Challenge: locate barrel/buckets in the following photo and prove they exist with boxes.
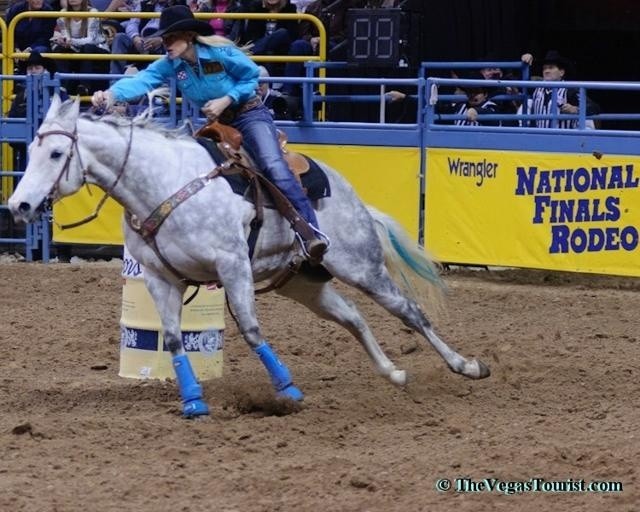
[119,247,225,380]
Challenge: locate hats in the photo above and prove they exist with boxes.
[535,48,566,66]
[17,51,56,70]
[101,19,126,33]
[144,3,215,40]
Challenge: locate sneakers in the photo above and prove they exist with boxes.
[305,236,325,267]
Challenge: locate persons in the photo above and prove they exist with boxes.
[87,4,332,268]
[1,0,595,136]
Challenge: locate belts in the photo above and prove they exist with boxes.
[220,96,261,119]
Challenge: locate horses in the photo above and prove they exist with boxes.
[7,88,491,420]
